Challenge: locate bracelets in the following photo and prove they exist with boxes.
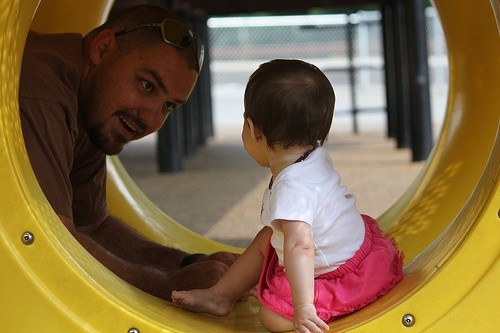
[181,252,208,268]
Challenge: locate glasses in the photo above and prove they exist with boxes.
[113,18,205,76]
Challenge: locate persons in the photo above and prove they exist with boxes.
[18,0,243,302]
[171,59,405,333]
